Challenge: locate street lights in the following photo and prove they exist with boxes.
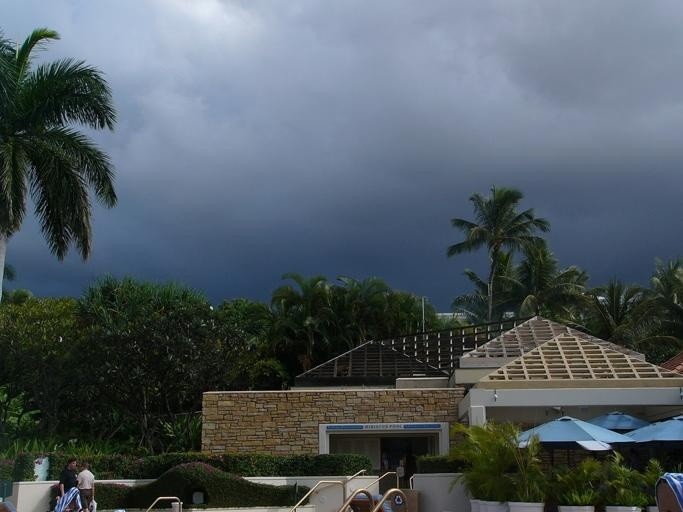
[89,500,97,512]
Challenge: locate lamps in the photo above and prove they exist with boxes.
[1,500,17,512]
[54,486,83,512]
[655,473,682,512]
[347,493,393,512]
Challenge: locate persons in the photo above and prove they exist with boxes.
[380,452,389,470]
[77,462,96,512]
[58,457,79,512]
[396,458,405,488]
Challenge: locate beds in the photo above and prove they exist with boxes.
[512,409,683,473]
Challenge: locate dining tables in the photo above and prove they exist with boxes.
[447,415,667,512]
